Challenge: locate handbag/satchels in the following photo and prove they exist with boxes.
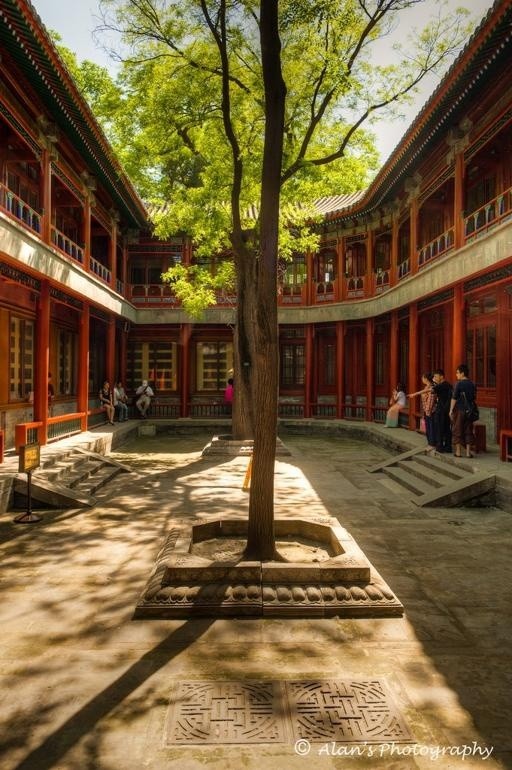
[463,401,479,422]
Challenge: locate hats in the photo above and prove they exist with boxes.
[142,380,148,386]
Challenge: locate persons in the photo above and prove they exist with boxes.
[222,379,234,411]
[135,381,155,417]
[96,380,115,424]
[47,371,56,417]
[113,380,131,422]
[405,364,480,460]
[383,381,407,430]
